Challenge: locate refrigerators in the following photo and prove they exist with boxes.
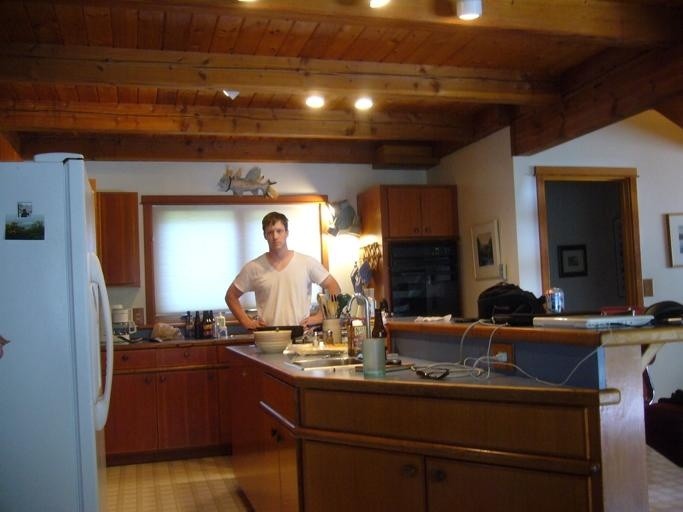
[0,160,112,508]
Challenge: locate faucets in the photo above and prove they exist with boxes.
[347,295,370,339]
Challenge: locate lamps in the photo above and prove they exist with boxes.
[456,1,482,22]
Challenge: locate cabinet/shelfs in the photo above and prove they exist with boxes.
[102,367,219,455]
[260,410,300,511]
[157,346,217,366]
[301,441,593,512]
[387,188,457,239]
[95,192,141,285]
[100,348,157,372]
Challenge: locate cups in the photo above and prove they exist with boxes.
[322,319,342,346]
[363,338,388,377]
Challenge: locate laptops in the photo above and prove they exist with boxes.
[532,313,657,331]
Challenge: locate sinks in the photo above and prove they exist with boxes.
[284,356,363,372]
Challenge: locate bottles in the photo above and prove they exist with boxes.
[202,310,214,338]
[325,330,334,346]
[195,311,201,339]
[312,332,319,347]
[371,308,389,362]
[184,311,193,339]
[545,286,564,313]
[217,326,227,339]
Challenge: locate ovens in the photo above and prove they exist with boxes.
[389,245,459,319]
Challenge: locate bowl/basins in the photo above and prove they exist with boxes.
[254,328,290,354]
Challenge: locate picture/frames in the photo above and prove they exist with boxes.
[470,217,502,281]
[558,245,587,278]
[666,213,683,267]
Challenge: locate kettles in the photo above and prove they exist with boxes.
[111,304,137,345]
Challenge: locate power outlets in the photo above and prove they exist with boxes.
[491,343,514,371]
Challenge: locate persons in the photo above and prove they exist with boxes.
[224,212,341,334]
